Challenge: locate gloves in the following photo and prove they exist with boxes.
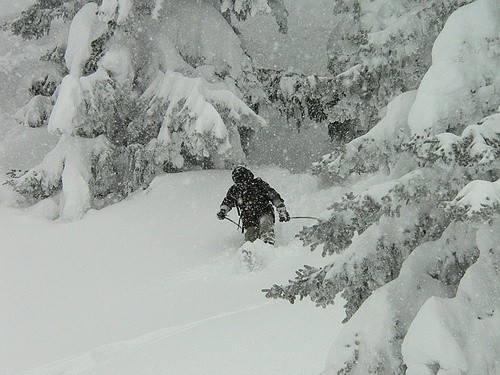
[276,207,290,222]
[217,204,231,220]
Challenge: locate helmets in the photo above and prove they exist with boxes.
[232,166,251,183]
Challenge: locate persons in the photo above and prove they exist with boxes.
[217,166,290,246]
